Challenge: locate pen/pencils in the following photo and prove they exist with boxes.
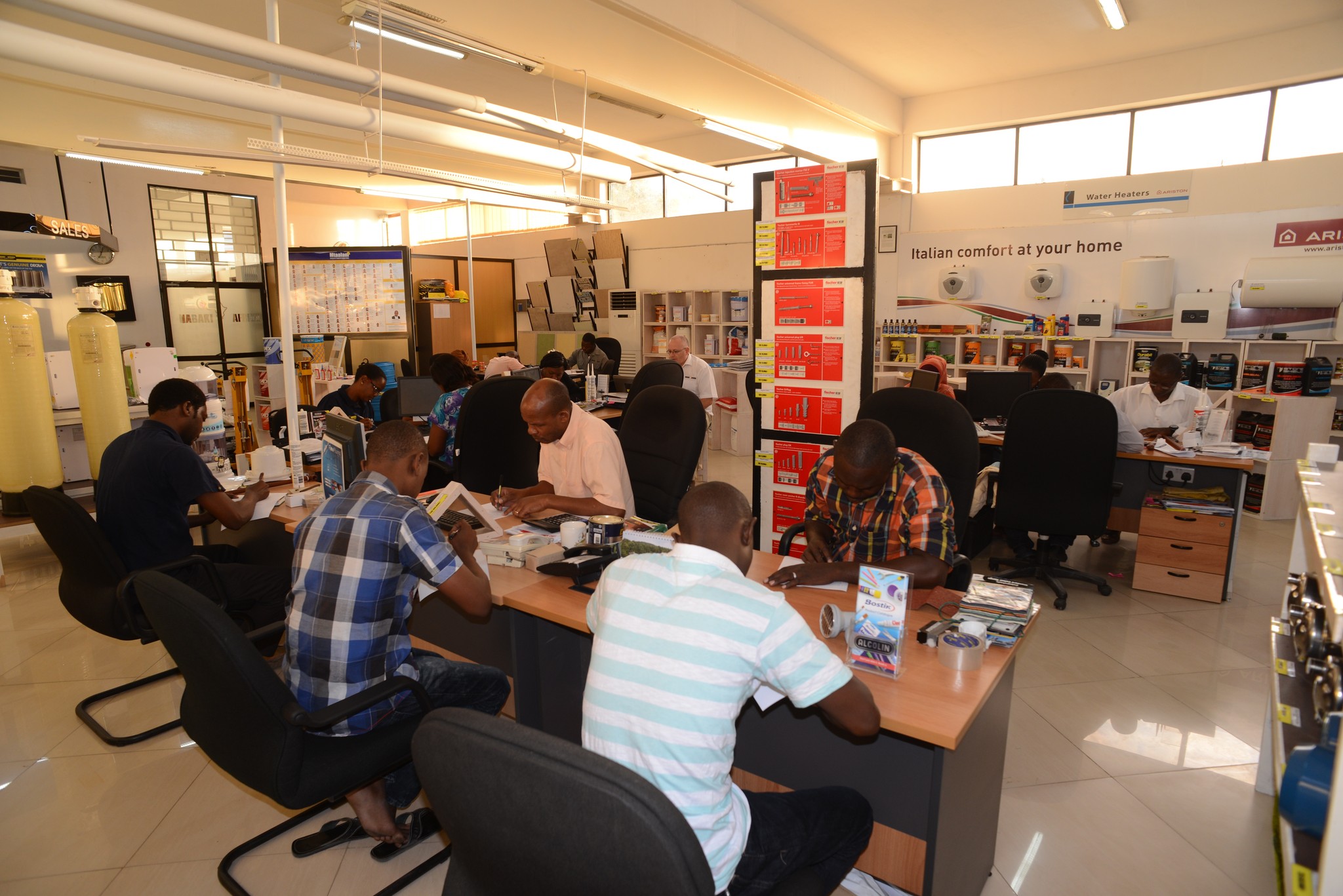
[448,527,462,540]
[496,485,501,512]
[528,364,532,368]
[259,472,264,481]
[353,412,375,427]
[820,537,836,560]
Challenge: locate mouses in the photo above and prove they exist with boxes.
[426,494,439,504]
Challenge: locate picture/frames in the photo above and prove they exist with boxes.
[878,224,897,253]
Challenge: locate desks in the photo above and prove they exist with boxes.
[284,488,589,720]
[196,474,327,548]
[314,374,356,407]
[973,423,1259,601]
[505,531,1043,896]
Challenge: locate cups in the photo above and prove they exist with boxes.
[560,521,586,550]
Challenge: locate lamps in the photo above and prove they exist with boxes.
[1096,0,1129,31]
[337,16,468,62]
[691,117,784,152]
[342,0,545,76]
[589,91,666,119]
[52,148,212,176]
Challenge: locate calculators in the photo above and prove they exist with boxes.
[521,513,589,534]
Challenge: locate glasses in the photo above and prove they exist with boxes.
[1146,368,1183,391]
[667,347,688,355]
[361,373,383,394]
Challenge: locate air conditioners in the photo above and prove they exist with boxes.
[608,287,643,377]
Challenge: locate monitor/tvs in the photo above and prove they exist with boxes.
[967,371,1032,431]
[613,375,635,393]
[321,413,367,501]
[510,366,542,381]
[397,375,444,417]
[910,368,940,391]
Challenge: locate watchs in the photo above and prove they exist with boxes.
[1169,425,1179,437]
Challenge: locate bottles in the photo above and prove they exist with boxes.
[585,363,591,402]
[590,363,596,401]
[883,319,918,333]
[321,364,325,380]
[315,364,320,379]
[327,365,332,380]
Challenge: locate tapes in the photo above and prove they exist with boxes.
[938,632,984,672]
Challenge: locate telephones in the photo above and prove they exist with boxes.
[537,545,620,586]
[479,532,550,568]
[974,421,989,437]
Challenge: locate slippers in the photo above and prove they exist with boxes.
[369,805,443,864]
[292,815,379,860]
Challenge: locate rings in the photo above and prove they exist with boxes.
[792,572,797,579]
[514,510,519,514]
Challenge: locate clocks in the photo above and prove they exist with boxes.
[88,242,115,265]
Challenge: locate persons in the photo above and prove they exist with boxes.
[1106,352,1213,451]
[764,419,957,593]
[451,348,480,386]
[993,372,1082,570]
[288,420,511,862]
[317,363,387,432]
[489,377,640,526]
[92,377,294,662]
[584,480,884,896]
[668,333,719,428]
[538,350,589,403]
[996,350,1050,424]
[904,355,957,402]
[484,351,529,382]
[564,332,609,389]
[424,353,482,470]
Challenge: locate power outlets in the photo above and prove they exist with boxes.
[1161,464,1195,484]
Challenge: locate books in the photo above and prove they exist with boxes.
[420,279,447,286]
[949,572,1035,649]
[1193,441,1244,460]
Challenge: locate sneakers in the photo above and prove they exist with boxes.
[687,480,697,491]
[1009,537,1036,560]
[1101,529,1122,544]
[1050,543,1068,563]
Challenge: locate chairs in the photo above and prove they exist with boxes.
[131,565,453,896]
[22,486,232,747]
[618,383,707,529]
[410,704,716,896]
[776,385,975,592]
[617,358,684,439]
[984,385,1120,610]
[574,337,622,401]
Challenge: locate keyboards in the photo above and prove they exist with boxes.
[414,501,485,533]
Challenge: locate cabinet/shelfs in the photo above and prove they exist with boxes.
[720,368,754,457]
[876,320,1343,462]
[705,366,731,450]
[526,233,630,329]
[254,397,286,432]
[415,300,473,377]
[250,362,286,399]
[641,285,755,367]
[1132,488,1233,604]
[1226,390,1338,521]
